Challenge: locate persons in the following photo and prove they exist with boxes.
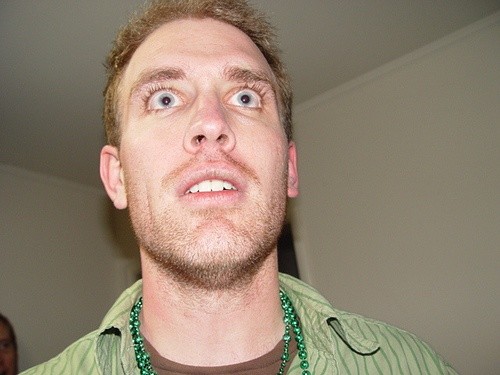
[0,0,465,375]
[0,311,19,375]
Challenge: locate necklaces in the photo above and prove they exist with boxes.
[130,289,312,375]
